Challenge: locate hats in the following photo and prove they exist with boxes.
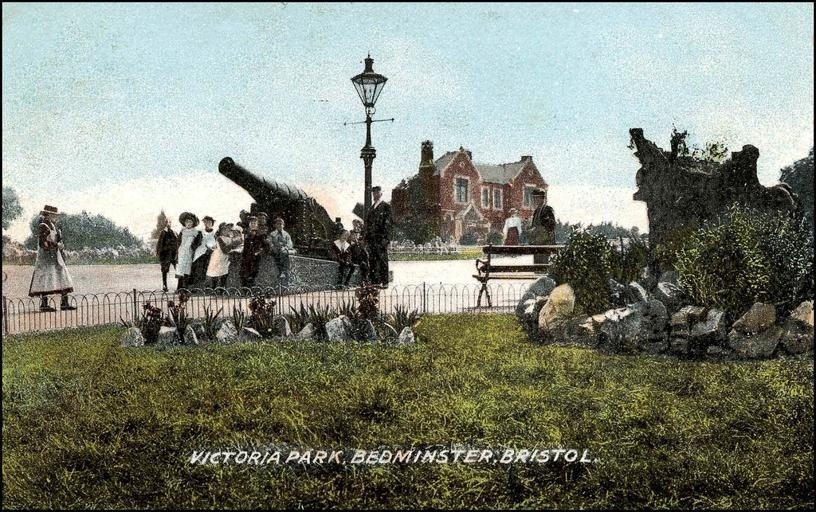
[248,215,258,221]
[531,188,546,197]
[202,215,216,222]
[217,222,233,236]
[179,211,199,228]
[41,204,63,216]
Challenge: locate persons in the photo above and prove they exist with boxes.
[26,204,79,313]
[530,188,556,274]
[502,207,526,244]
[365,185,393,289]
[154,211,363,300]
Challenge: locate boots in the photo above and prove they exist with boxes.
[40,296,57,312]
[60,295,76,310]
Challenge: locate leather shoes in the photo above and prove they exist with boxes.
[382,283,390,289]
[372,284,380,288]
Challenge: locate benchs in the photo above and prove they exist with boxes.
[472,244,567,311]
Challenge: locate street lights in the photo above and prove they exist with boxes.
[344,49,394,326]
